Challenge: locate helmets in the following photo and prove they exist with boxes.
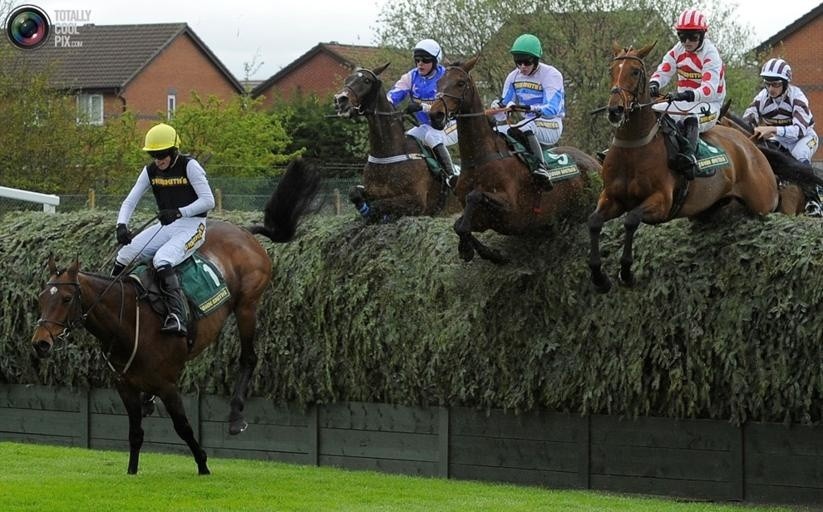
[676,10,707,32]
[142,123,180,151]
[410,39,442,65]
[760,57,792,82]
[509,34,542,58]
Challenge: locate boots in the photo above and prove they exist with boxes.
[432,142,458,187]
[157,264,188,336]
[523,134,553,192]
[676,125,699,180]
[799,167,823,212]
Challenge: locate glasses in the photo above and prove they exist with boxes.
[678,32,699,42]
[515,59,534,66]
[413,56,435,63]
[148,147,175,159]
[763,78,783,87]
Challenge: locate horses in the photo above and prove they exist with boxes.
[586,39,780,294]
[31,156,330,475]
[333,62,465,225]
[716,99,823,218]
[429,53,604,268]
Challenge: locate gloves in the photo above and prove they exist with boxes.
[407,102,422,114]
[492,101,504,111]
[649,81,660,97]
[116,223,131,245]
[158,208,182,225]
[669,91,689,102]
[506,101,518,110]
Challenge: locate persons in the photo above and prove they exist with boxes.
[386,41,461,191]
[743,58,822,218]
[490,33,567,192]
[112,121,216,338]
[647,8,729,181]
